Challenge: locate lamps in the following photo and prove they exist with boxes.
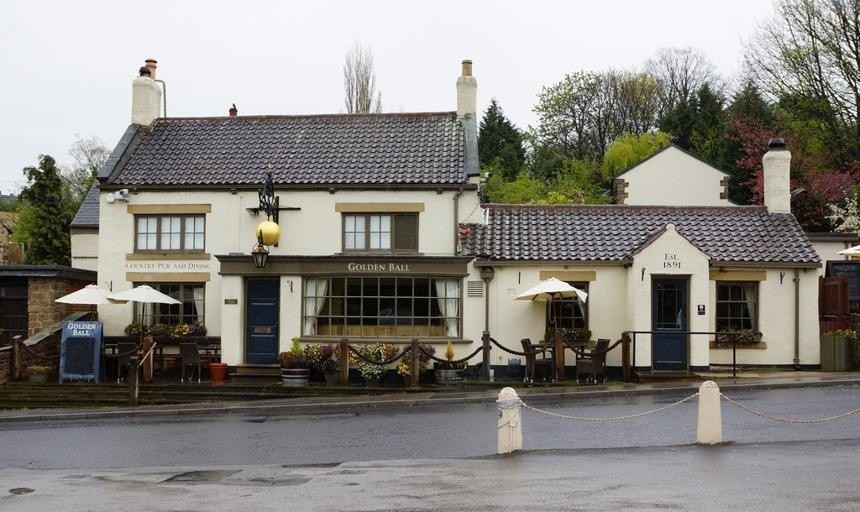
[250,229,270,267]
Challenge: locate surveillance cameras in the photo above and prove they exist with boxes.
[119,189,128,198]
[485,172,493,178]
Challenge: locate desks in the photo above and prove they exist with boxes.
[197,346,221,383]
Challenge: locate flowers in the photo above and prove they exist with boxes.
[304,343,435,385]
[823,329,858,344]
[718,328,762,345]
[124,320,207,342]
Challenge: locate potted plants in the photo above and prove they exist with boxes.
[26,364,56,382]
[433,361,467,385]
[277,337,311,387]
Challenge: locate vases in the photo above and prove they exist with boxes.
[820,336,857,372]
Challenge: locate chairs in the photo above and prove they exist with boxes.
[521,338,610,388]
[101,343,208,385]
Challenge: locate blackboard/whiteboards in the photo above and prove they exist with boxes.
[59,321,102,380]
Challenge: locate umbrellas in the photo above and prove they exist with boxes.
[105,284,183,345]
[54,282,130,321]
[514,275,589,330]
